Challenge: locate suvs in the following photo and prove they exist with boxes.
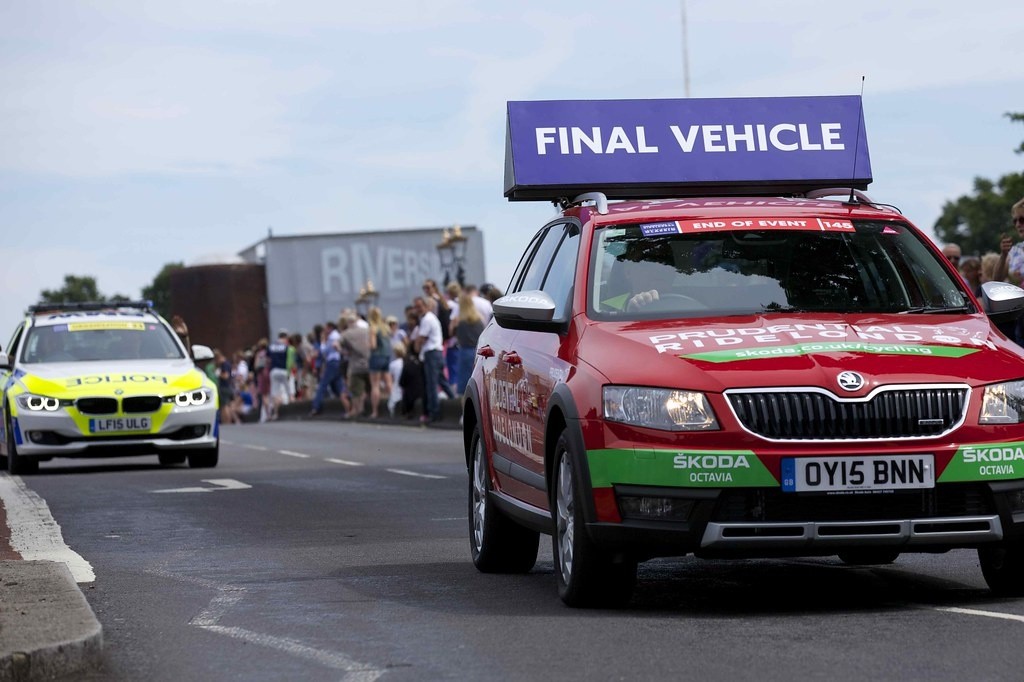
[460,186,1021,609]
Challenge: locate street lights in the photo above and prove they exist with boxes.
[436,225,468,289]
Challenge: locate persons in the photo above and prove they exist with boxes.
[388,278,505,424]
[942,196,1024,299]
[214,308,394,426]
[601,245,677,315]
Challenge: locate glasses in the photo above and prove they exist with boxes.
[1012,215,1024,225]
[422,284,434,289]
[947,255,960,261]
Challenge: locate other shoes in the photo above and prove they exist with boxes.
[263,386,463,428]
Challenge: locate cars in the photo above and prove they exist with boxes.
[0,301,223,473]
